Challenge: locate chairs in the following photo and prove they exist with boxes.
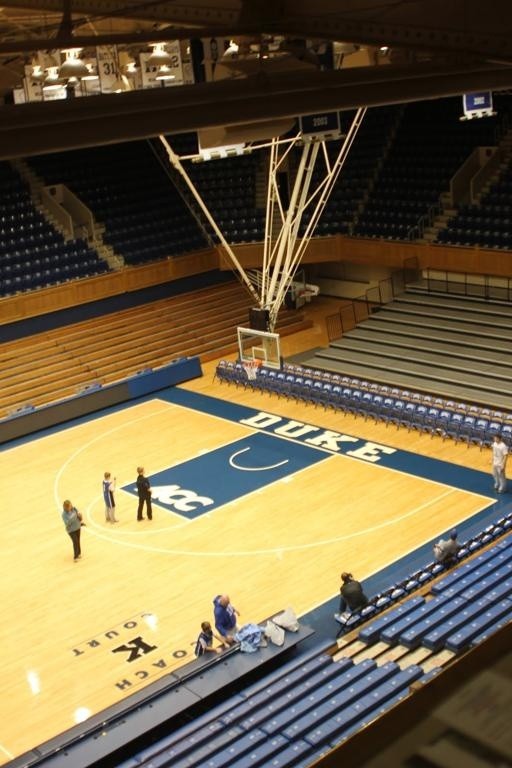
[334,508,512,636]
[212,357,511,452]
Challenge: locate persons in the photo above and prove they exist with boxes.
[213,595,241,645]
[103,472,117,521]
[284,279,295,310]
[276,176,288,211]
[137,469,152,520]
[434,528,464,562]
[333,573,367,619]
[63,501,85,559]
[490,434,508,492]
[196,622,230,657]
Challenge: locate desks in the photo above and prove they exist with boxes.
[0,610,315,767]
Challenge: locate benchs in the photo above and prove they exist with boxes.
[299,271,512,413]
